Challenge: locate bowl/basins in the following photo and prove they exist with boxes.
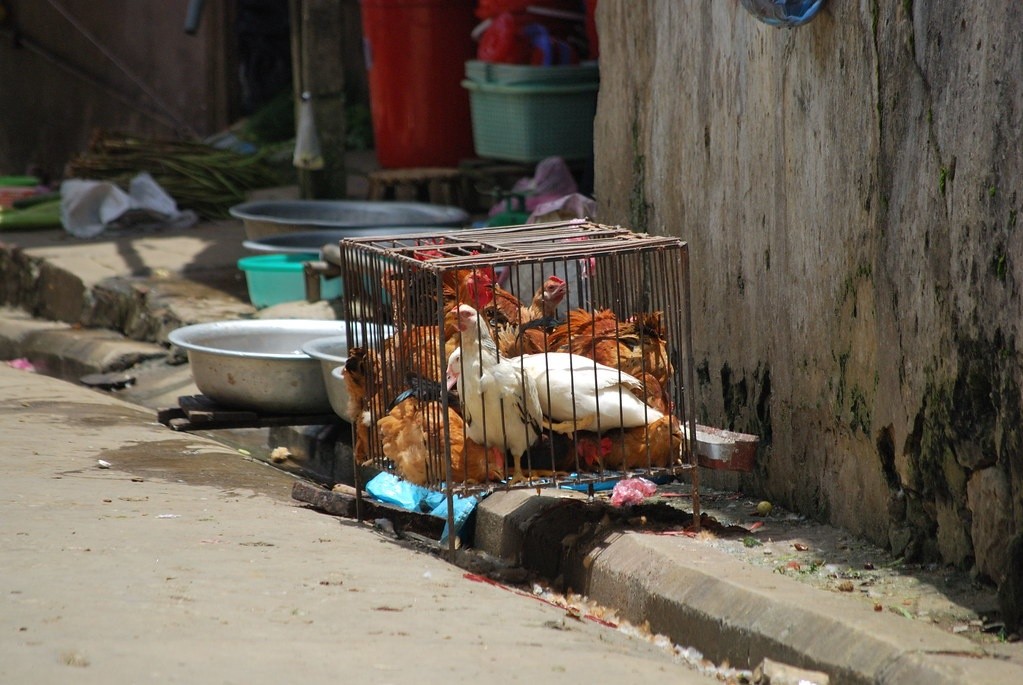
[228,201,469,241]
[237,253,393,311]
[242,226,462,255]
[303,335,393,423]
[167,318,394,411]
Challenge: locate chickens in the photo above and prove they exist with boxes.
[344,238,683,482]
